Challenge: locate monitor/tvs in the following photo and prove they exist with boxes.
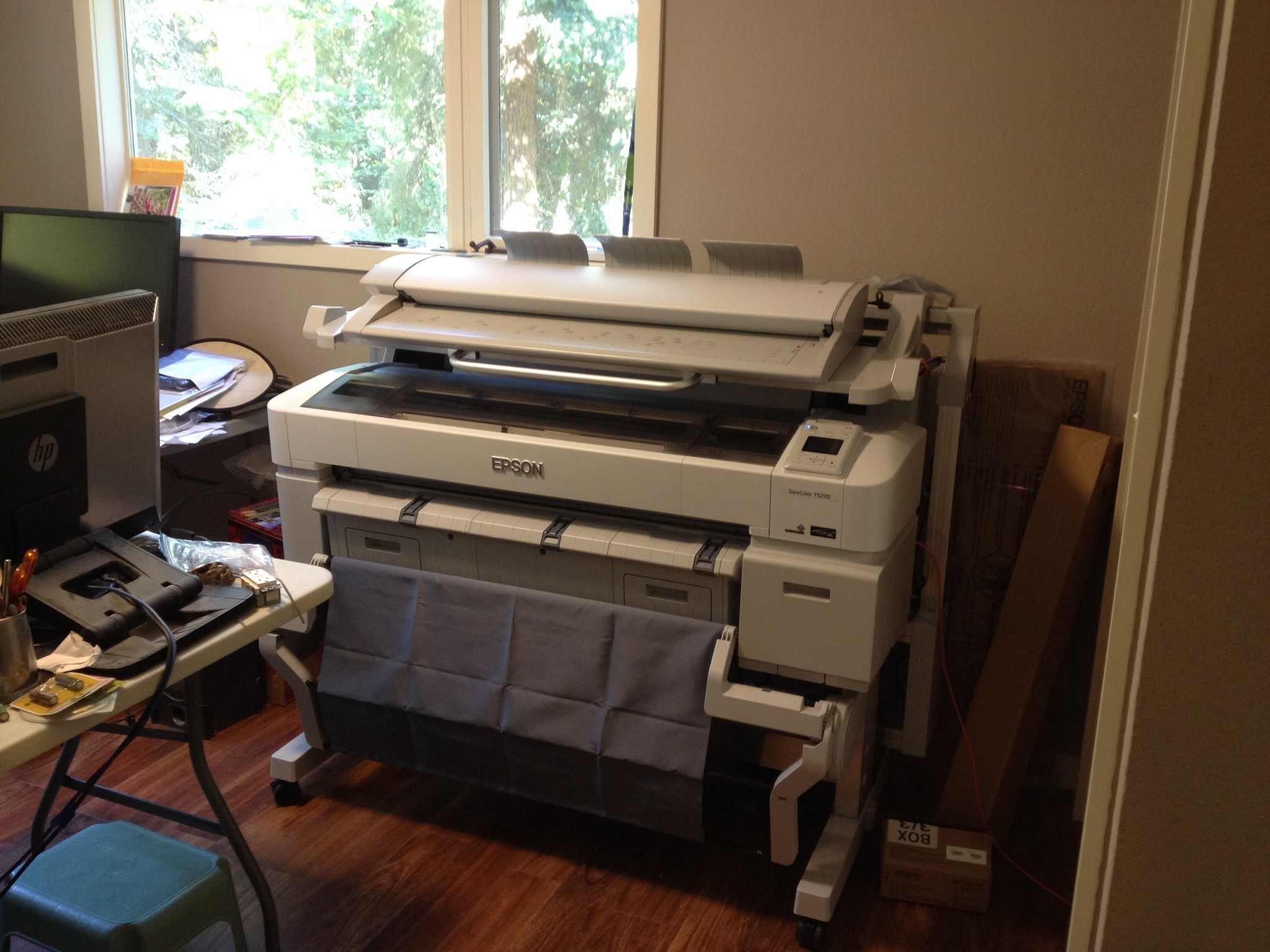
[1,288,253,683]
[0,205,180,354]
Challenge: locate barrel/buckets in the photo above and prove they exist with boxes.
[0,606,39,704]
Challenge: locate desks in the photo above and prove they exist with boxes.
[0,558,334,952]
[160,406,268,488]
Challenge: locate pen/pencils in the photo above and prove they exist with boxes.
[1,558,11,617]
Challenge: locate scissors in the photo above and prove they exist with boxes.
[9,547,38,607]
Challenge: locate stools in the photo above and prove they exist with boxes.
[0,821,248,952]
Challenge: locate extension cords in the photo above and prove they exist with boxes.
[243,570,277,587]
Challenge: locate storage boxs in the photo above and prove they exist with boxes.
[227,497,283,560]
[880,363,1117,913]
